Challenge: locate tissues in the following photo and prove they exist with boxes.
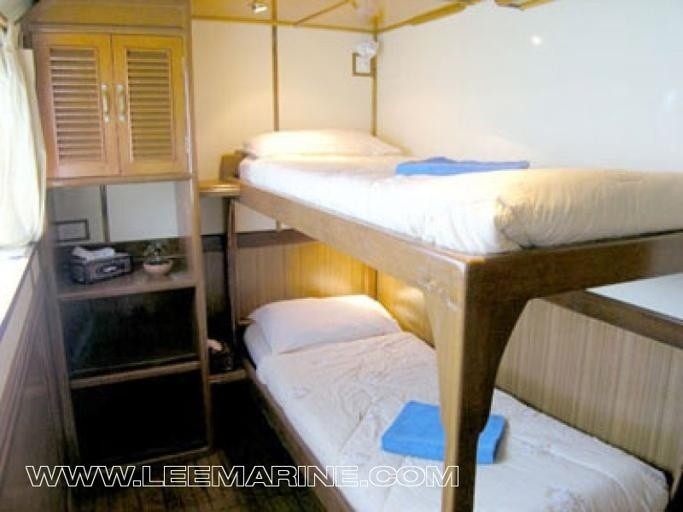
[69,246,133,283]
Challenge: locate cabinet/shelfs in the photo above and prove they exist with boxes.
[28,22,215,478]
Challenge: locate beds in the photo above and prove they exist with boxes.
[219,147,683,512]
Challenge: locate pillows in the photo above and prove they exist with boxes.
[246,294,402,354]
[243,129,404,156]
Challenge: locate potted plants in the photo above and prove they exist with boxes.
[142,241,173,275]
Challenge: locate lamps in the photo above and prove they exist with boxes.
[252,0,268,13]
[352,39,379,76]
